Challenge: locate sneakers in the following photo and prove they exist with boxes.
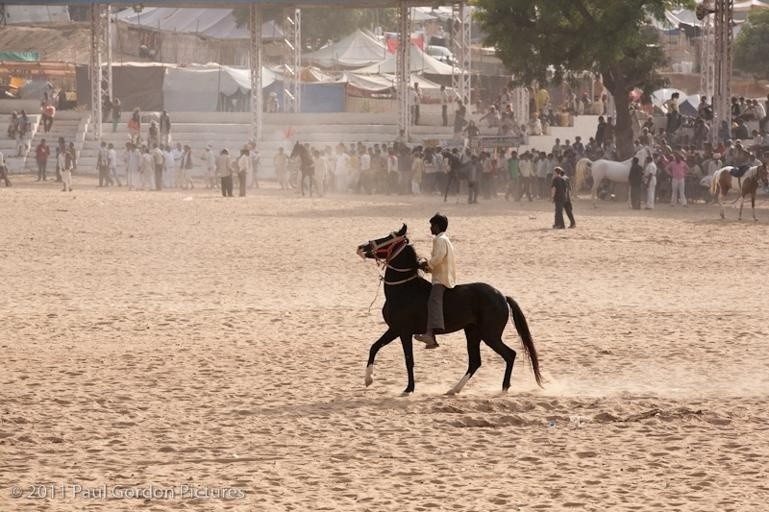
[552,224,566,230]
[425,343,440,349]
[567,223,576,229]
[415,334,436,345]
[410,189,551,203]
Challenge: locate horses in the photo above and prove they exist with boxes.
[355,222,546,398]
[575,145,655,209]
[289,140,330,198]
[440,151,483,205]
[711,160,769,221]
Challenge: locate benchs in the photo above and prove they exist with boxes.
[0,112,454,179]
[463,112,527,148]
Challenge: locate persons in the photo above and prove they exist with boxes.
[414,213,455,349]
